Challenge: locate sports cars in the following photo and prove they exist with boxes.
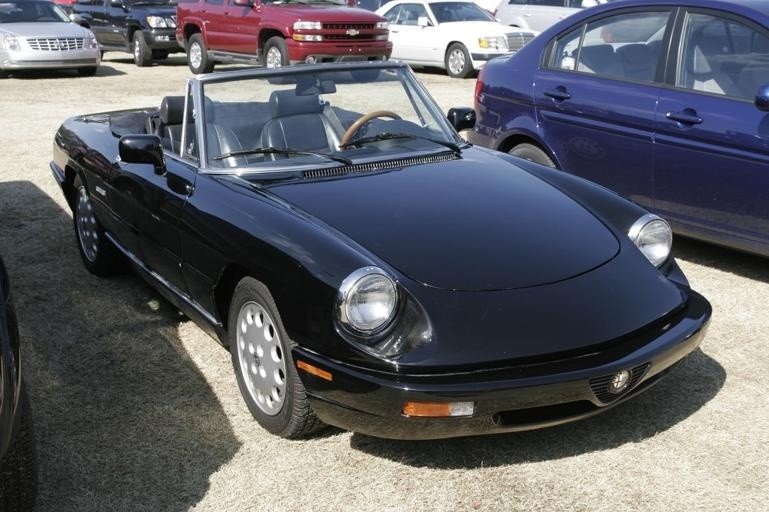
[49,61,712,442]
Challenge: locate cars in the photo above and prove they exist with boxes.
[474,0,769,259]
[1,247,23,469]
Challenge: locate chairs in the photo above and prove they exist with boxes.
[648,39,662,57]
[618,41,652,86]
[689,35,739,95]
[265,91,333,153]
[575,41,625,77]
[161,94,245,164]
